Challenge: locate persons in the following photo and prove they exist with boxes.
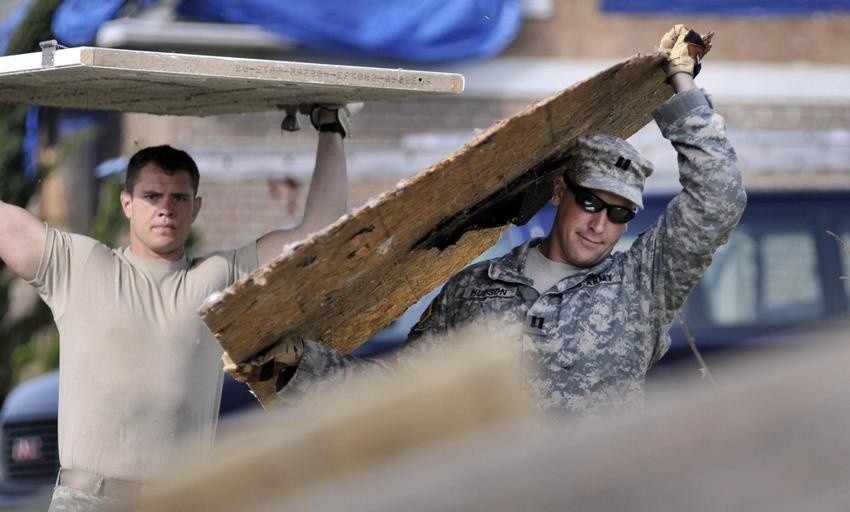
[1,101,365,512]
[219,22,749,426]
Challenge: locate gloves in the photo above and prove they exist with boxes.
[659,23,704,83]
[308,98,365,138]
[220,336,304,385]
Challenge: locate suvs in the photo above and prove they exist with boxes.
[1,169,850,509]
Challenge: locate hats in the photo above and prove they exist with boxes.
[565,131,653,211]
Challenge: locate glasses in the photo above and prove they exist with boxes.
[566,181,637,224]
